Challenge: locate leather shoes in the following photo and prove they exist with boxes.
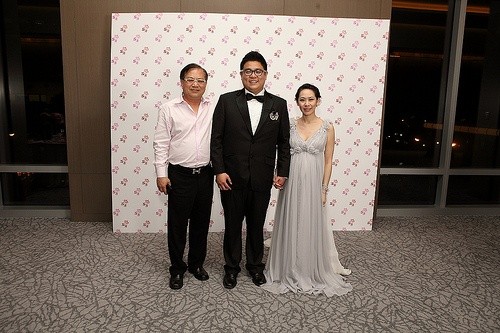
[223,272,238,289]
[170,272,183,289]
[187,266,209,280]
[246,271,266,286]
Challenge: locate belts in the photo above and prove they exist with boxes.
[169,163,211,174]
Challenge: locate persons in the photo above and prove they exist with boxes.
[209,51,291,289]
[153,63,213,289]
[261,83,352,298]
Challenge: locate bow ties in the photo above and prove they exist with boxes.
[247,93,264,103]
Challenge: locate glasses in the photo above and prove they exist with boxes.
[240,68,266,76]
[184,78,206,84]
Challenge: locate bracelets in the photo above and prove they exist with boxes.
[322,184,329,191]
